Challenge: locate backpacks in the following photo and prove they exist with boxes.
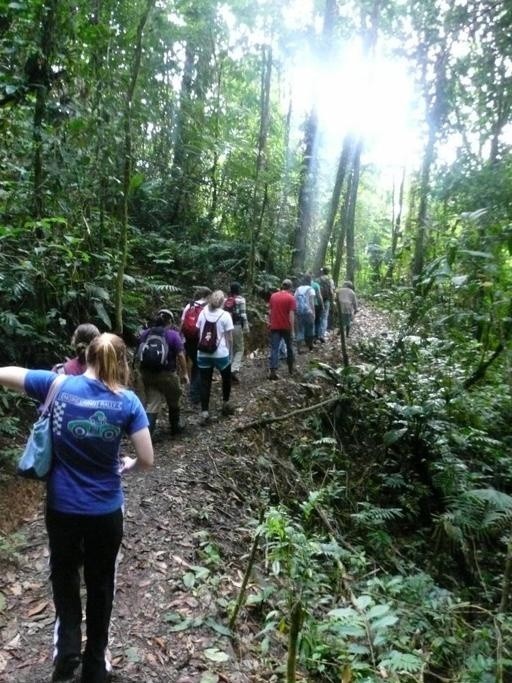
[222,295,241,321]
[196,309,224,353]
[182,301,208,339]
[140,324,169,371]
[319,276,333,300]
[295,286,313,316]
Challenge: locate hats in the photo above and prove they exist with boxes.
[282,278,292,288]
[230,281,244,289]
[343,280,353,286]
[322,266,330,271]
[156,308,177,323]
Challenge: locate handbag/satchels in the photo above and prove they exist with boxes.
[16,416,53,481]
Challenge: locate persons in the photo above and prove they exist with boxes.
[46,322,100,377]
[137,264,359,439]
[1,330,158,681]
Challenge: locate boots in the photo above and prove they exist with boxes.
[168,405,182,435]
[336,325,351,337]
[146,412,158,444]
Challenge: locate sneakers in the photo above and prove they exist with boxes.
[268,368,279,380]
[299,337,325,354]
[289,365,297,374]
[232,371,240,383]
[223,405,234,416]
[52,656,117,681]
[200,414,212,426]
[118,453,138,474]
